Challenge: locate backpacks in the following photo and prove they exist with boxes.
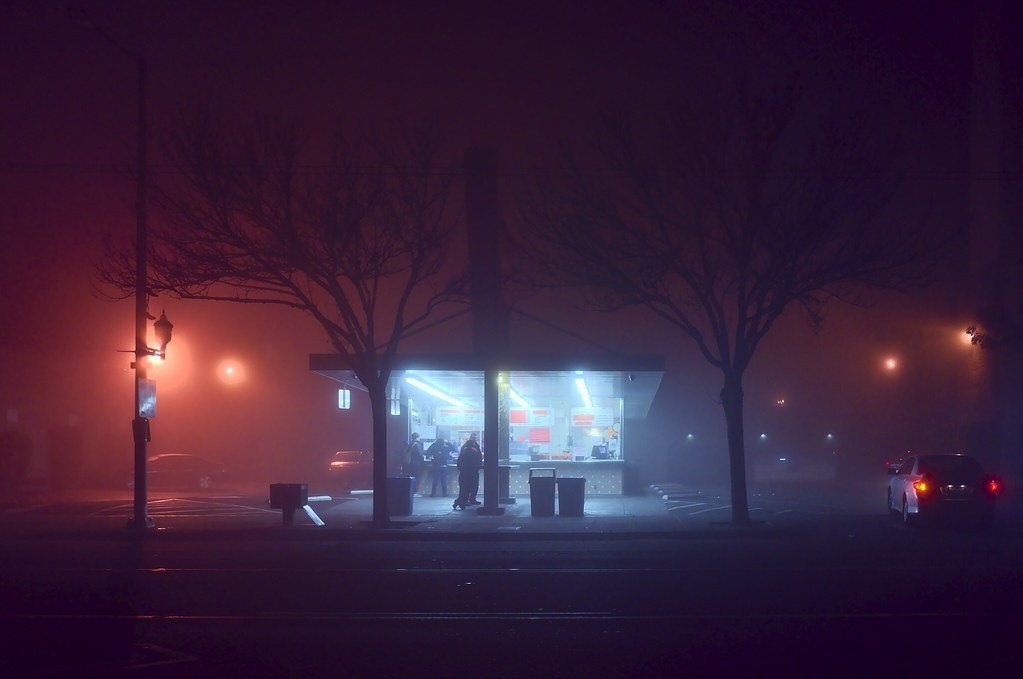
[402,442,417,464]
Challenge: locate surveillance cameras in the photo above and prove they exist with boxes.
[629,376,635,381]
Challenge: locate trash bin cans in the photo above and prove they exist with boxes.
[526,467,557,518]
[555,476,587,520]
[385,475,415,517]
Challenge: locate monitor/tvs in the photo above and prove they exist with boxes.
[591,445,607,459]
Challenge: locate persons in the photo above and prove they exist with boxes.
[447,440,458,460]
[452,440,480,510]
[426,437,454,497]
[406,433,424,497]
[460,433,483,506]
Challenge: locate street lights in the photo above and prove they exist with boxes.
[133,305,171,514]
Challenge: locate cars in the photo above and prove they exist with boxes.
[887,454,1001,524]
[327,450,373,485]
[116,454,228,491]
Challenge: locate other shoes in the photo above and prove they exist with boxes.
[461,506,466,510]
[413,494,422,498]
[469,498,481,505]
[453,500,458,508]
[465,502,472,506]
